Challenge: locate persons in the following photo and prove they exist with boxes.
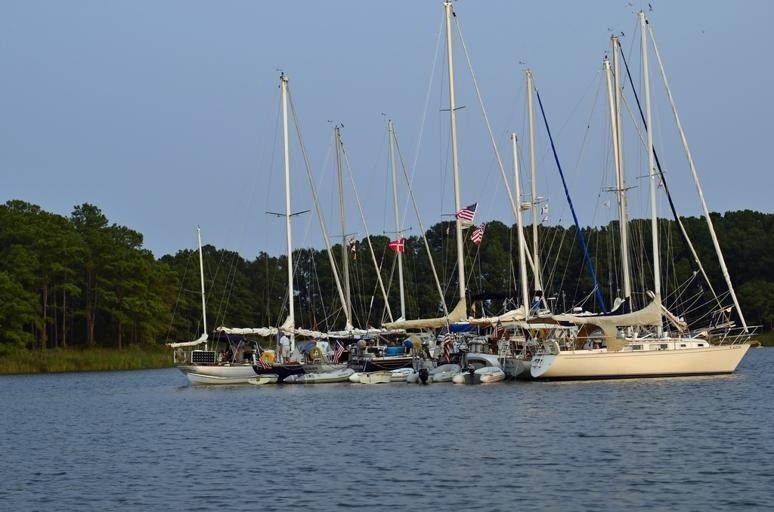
[278,331,290,365]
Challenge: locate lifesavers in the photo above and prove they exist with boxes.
[310,347,322,359]
[544,340,560,355]
[262,352,273,363]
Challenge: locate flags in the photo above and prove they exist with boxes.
[388,238,403,252]
[490,320,503,341]
[442,325,451,363]
[454,202,476,223]
[258,357,271,369]
[467,222,486,245]
[331,341,344,365]
